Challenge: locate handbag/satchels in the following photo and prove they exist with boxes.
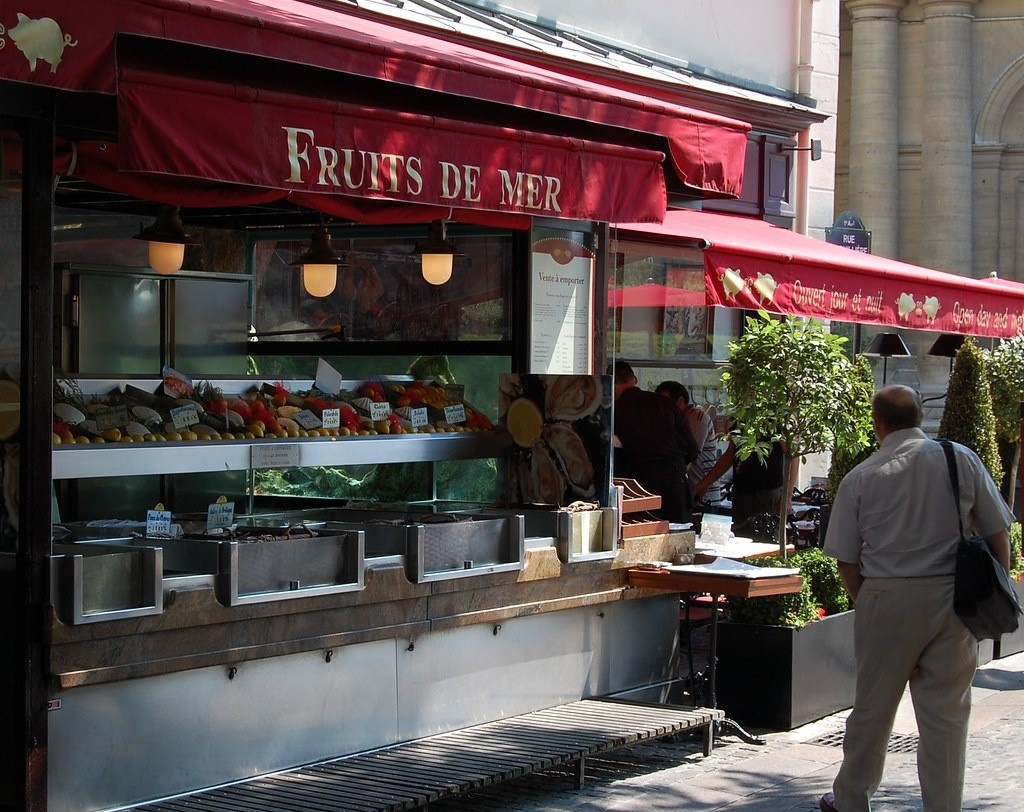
[952,538,1024,641]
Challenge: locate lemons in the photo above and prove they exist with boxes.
[506,398,543,447]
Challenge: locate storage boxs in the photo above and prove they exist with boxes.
[50,505,621,628]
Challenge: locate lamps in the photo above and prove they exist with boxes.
[863,334,966,405]
[783,138,822,161]
[288,211,352,299]
[408,216,469,286]
[133,203,202,277]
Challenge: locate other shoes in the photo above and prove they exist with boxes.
[819,793,837,812]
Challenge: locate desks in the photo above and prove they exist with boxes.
[628,533,804,745]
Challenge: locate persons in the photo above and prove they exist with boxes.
[819,382,1016,812]
[606,360,800,525]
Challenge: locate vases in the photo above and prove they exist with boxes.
[975,572,1024,668]
[712,608,860,732]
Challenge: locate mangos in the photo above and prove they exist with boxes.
[52,381,487,445]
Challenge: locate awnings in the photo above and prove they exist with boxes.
[0,0,753,232]
[593,206,1024,339]
